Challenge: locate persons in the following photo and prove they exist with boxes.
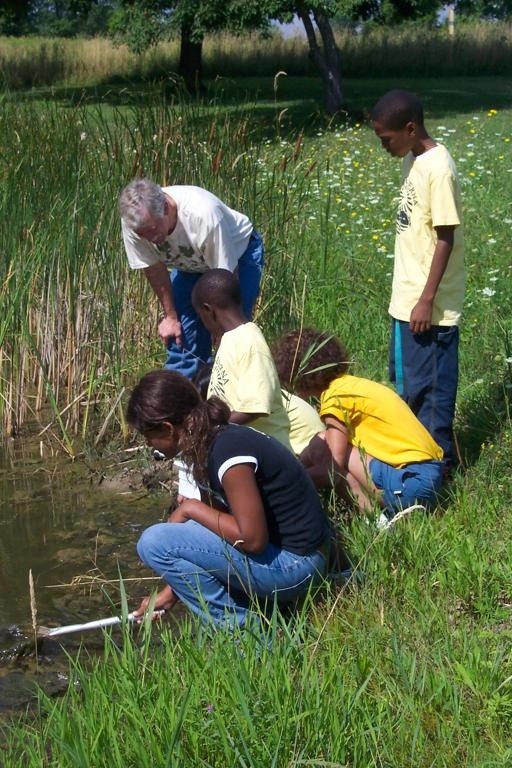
[278,389,324,454]
[371,88,464,462]
[192,267,290,452]
[122,181,264,459]
[275,327,444,531]
[132,371,332,661]
